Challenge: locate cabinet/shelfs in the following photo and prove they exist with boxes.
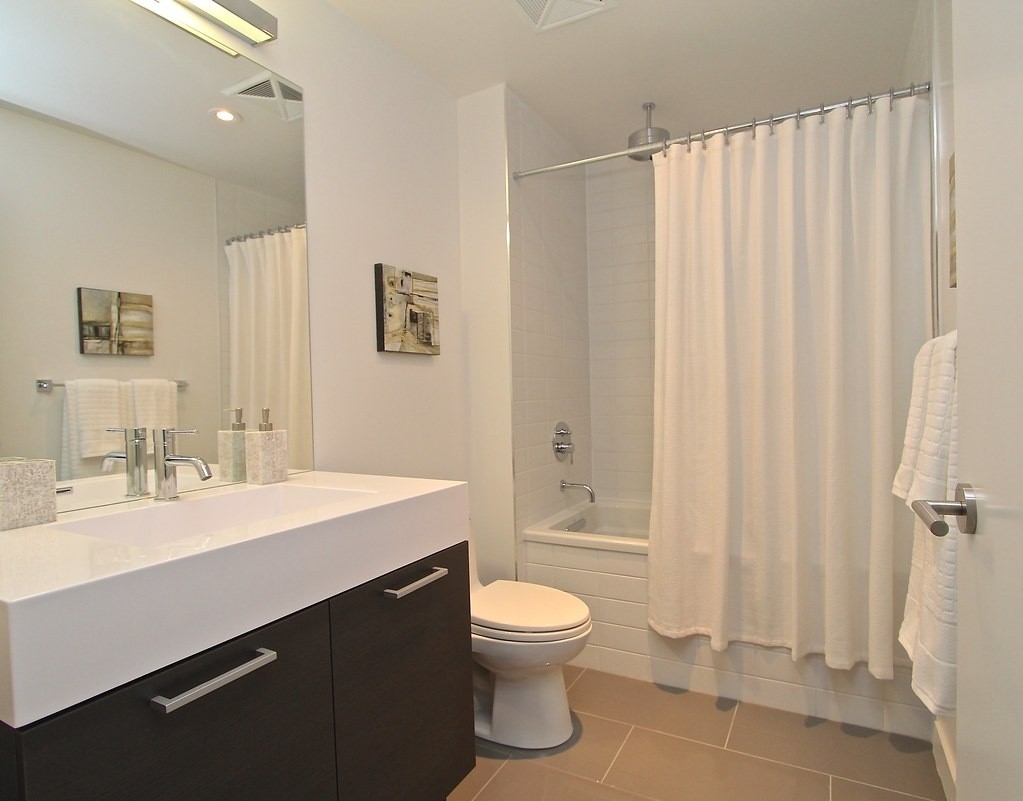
[0,538,477,801]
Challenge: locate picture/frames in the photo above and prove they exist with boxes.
[374,262,444,359]
[75,285,159,360]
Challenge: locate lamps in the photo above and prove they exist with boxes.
[174,0,279,47]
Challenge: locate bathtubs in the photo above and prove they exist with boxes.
[519,495,935,745]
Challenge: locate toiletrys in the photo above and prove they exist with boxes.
[217,405,253,485]
[243,407,289,488]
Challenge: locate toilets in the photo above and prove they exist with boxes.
[467,543,595,751]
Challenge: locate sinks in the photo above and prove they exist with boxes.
[0,469,474,733]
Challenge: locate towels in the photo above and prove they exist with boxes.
[889,335,950,501]
[897,503,922,665]
[61,378,181,489]
[910,332,957,719]
[904,328,960,516]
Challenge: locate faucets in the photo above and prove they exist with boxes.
[97,425,155,498]
[152,426,213,499]
[559,478,596,504]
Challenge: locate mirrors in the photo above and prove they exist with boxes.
[0,0,312,522]
